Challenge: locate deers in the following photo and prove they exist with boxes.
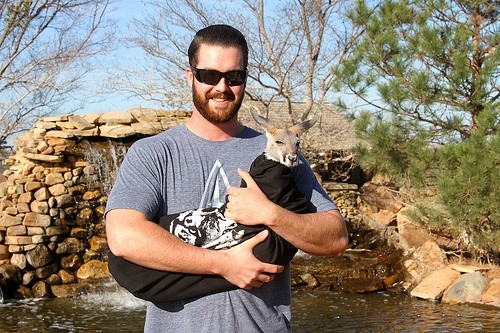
[249,106,320,168]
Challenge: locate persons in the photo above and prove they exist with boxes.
[103,24,349,332]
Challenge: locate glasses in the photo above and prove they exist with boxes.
[188,65,249,88]
